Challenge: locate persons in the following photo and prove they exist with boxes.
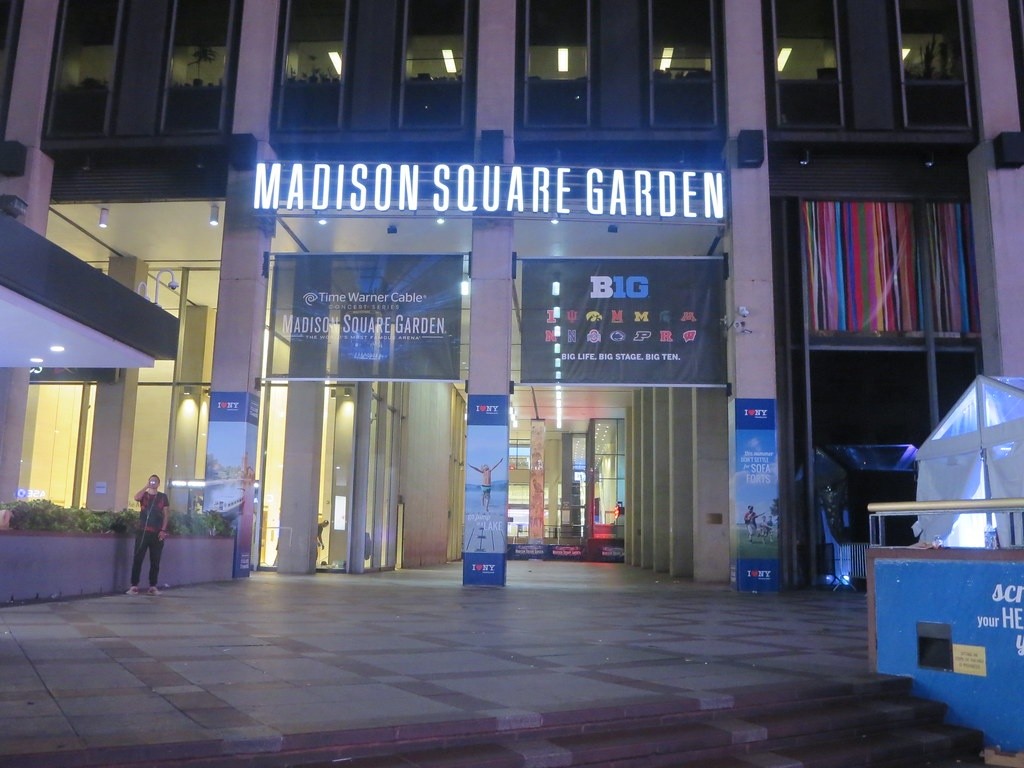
[759,516,774,548]
[317,520,329,551]
[125,474,169,595]
[744,505,758,542]
[468,458,502,513]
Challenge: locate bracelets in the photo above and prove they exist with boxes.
[160,528,166,533]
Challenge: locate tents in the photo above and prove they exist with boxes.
[913,371,1024,549]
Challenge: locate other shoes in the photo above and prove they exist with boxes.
[148,586,161,595]
[126,586,138,595]
